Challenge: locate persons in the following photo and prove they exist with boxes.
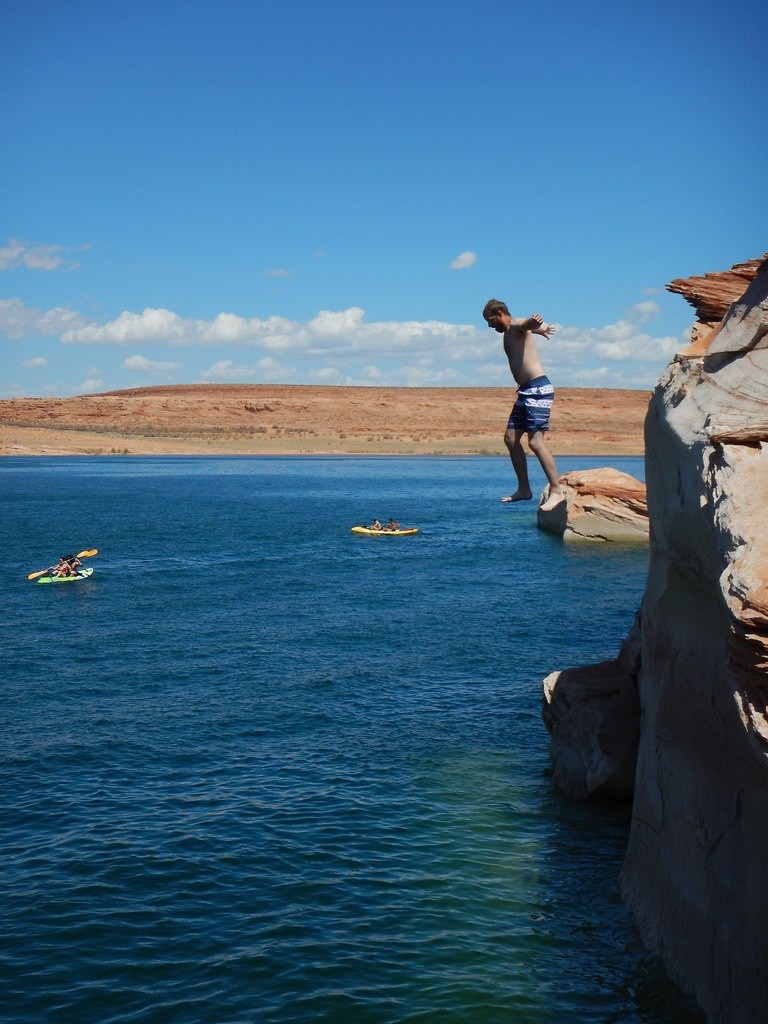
[367,519,396,531]
[53,555,83,576]
[484,298,566,513]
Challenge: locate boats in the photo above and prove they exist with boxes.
[37,568,94,584]
[351,526,419,535]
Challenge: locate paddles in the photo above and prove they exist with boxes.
[27,550,88,580]
[86,548,98,558]
